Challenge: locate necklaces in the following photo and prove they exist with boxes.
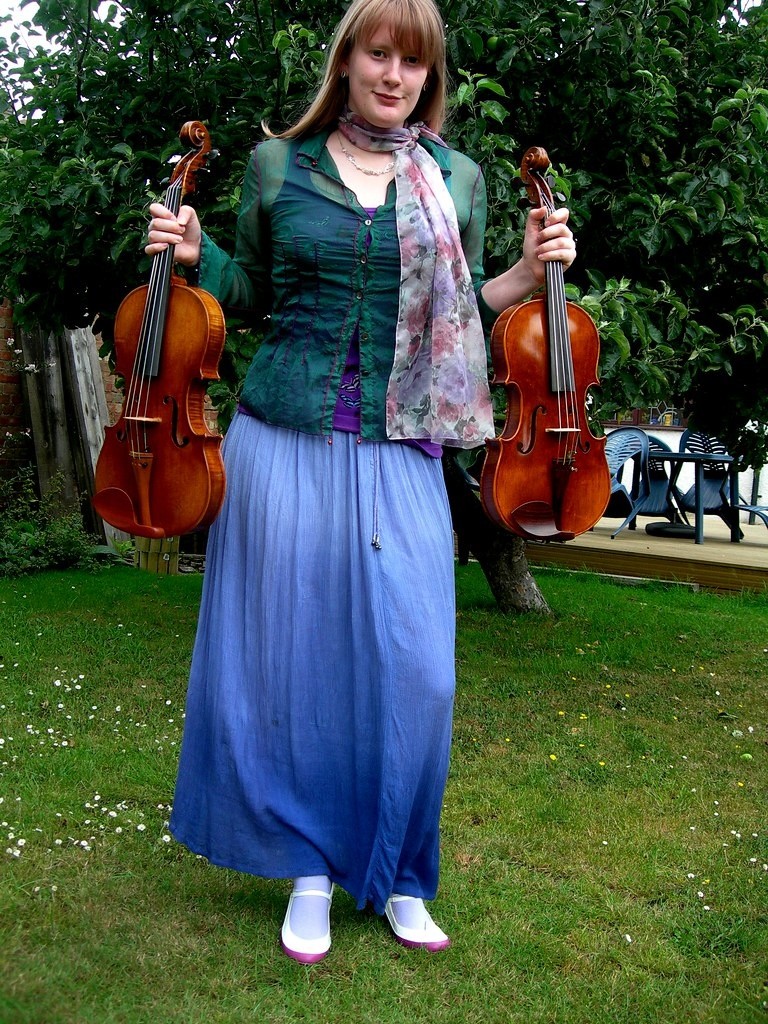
[337,130,395,178]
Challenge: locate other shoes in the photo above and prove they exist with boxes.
[385,893,451,952]
[279,881,335,964]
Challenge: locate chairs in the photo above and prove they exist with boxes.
[603,427,768,539]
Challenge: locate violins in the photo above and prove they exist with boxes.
[479,146,612,542]
[91,121,227,541]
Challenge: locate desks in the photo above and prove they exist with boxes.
[628,451,743,544]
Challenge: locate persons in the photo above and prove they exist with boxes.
[145,0,575,966]
